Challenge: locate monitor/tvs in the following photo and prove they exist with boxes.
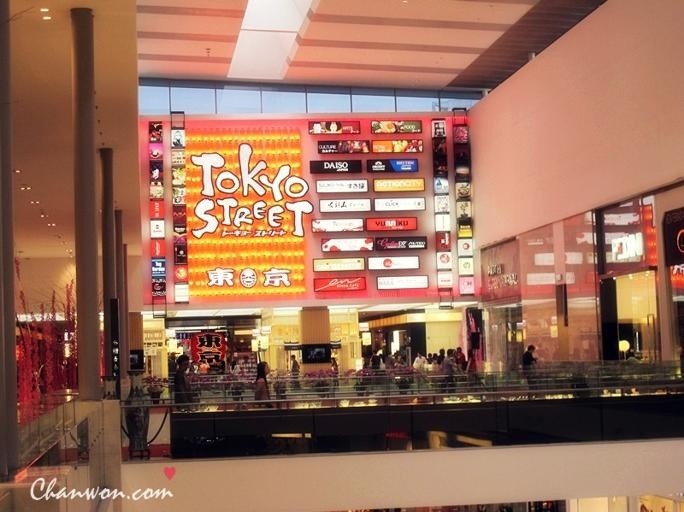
[302,344,331,363]
[130,350,143,369]
[466,309,482,333]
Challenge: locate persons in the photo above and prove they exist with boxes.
[254,360,274,408]
[361,346,477,395]
[329,357,340,391]
[166,351,242,386]
[522,344,539,390]
[173,355,196,411]
[289,354,302,391]
